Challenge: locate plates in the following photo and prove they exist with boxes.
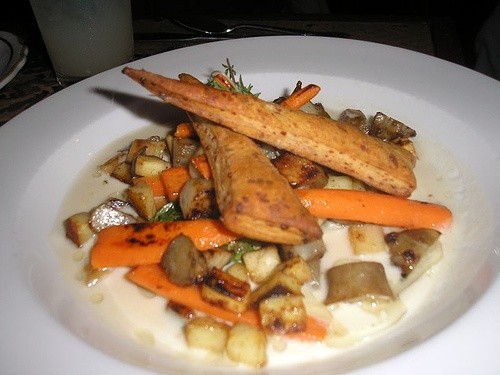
[0,36,500,375]
[0,31,28,90]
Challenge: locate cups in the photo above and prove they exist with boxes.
[31,0,134,89]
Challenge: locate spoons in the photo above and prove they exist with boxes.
[168,11,350,39]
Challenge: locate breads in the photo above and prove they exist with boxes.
[122,65,416,245]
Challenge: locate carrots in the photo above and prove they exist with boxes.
[88,84,452,344]
